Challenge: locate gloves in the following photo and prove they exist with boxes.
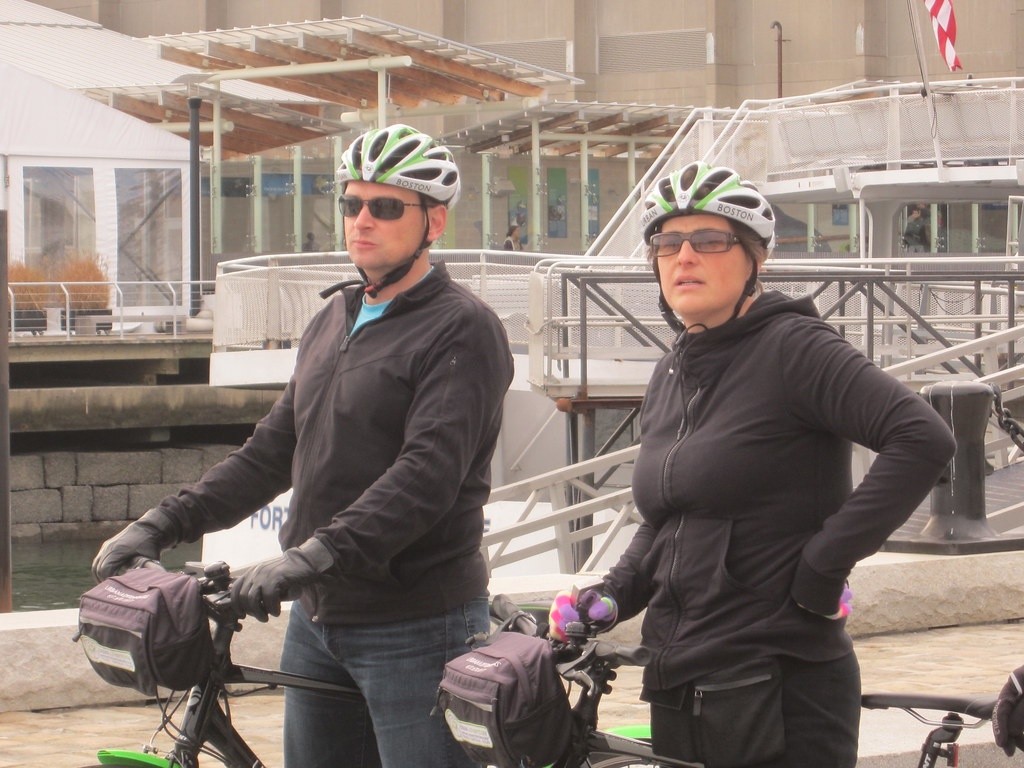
[796,587,852,620]
[91,507,180,585]
[228,547,322,624]
[992,663,1024,757]
[547,585,617,643]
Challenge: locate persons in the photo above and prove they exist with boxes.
[89,123,515,768]
[903,209,927,253]
[548,160,956,768]
[504,224,524,251]
[302,231,320,252]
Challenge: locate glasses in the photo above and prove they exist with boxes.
[649,230,740,257]
[336,194,423,221]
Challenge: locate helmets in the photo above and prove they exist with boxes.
[640,161,775,263]
[336,124,461,204]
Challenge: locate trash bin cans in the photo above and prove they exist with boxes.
[75,308,113,337]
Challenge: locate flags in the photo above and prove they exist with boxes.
[922,0,962,71]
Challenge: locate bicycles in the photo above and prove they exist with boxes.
[432,594,709,768]
[860,693,1024,767]
[71,556,363,768]
